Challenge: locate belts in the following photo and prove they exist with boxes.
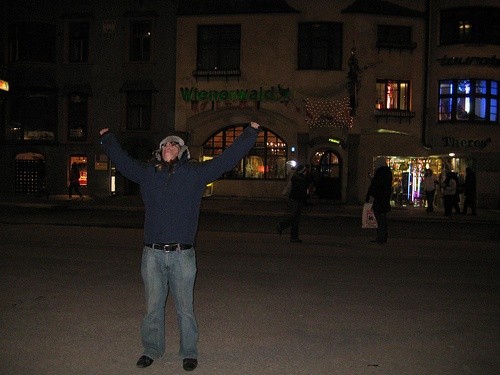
[145,243,192,253]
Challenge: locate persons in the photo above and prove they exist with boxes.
[441,166,476,217]
[422,169,436,213]
[275,164,314,243]
[99,121,259,371]
[67,162,84,201]
[366,157,393,246]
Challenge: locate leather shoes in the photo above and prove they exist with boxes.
[183,358,197,370]
[136,356,153,367]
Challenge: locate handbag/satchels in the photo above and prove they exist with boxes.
[361,203,378,228]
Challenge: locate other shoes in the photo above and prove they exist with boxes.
[275,223,281,234]
[290,238,302,243]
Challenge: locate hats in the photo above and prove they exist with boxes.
[296,164,306,173]
[152,136,190,162]
